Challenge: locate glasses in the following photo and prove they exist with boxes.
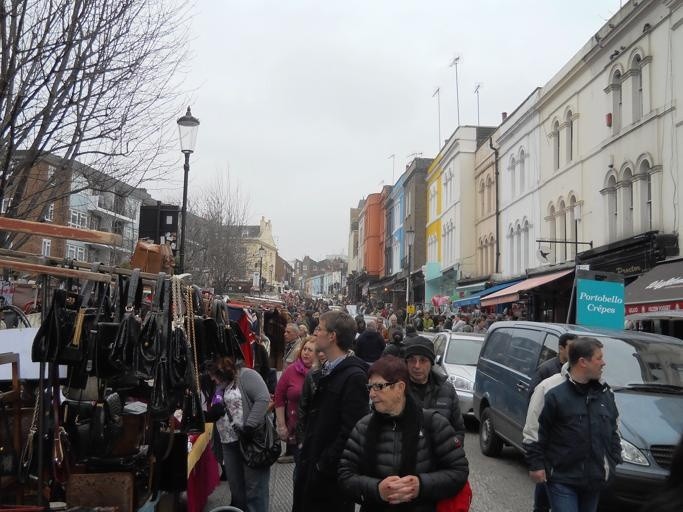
[407,357,431,363]
[316,326,336,333]
[367,382,393,391]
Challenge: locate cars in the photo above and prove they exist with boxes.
[429,328,488,423]
[316,293,391,342]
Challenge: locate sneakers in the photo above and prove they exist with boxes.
[277,455,294,464]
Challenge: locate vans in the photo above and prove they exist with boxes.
[470,316,683,511]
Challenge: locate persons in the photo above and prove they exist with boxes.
[292,310,371,512]
[282,323,302,371]
[274,335,326,483]
[382,331,409,357]
[401,336,466,447]
[336,355,469,512]
[355,321,386,363]
[406,310,505,336]
[522,336,622,512]
[206,355,271,512]
[286,294,406,338]
[527,333,579,512]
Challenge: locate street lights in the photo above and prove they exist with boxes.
[176,103,200,274]
[256,243,264,297]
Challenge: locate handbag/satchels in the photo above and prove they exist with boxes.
[435,479,473,512]
[238,417,282,470]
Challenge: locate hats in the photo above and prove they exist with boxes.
[405,335,436,365]
